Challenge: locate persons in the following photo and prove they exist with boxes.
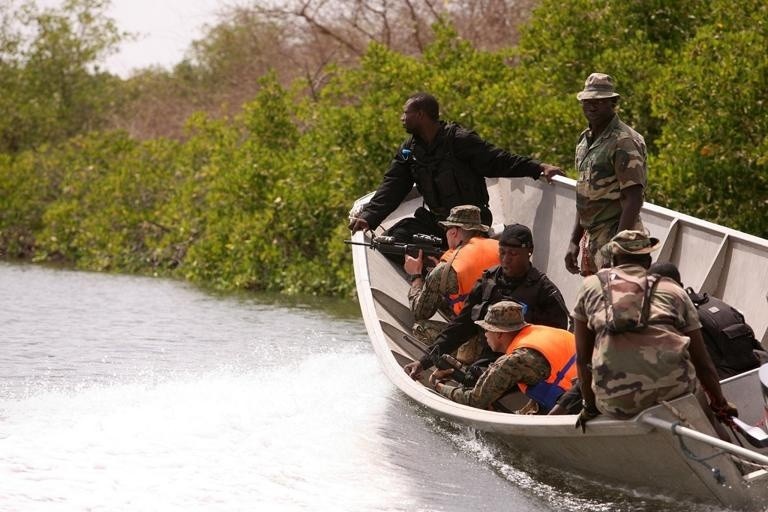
[565,73,648,276]
[404,204,501,344]
[347,94,566,262]
[404,224,567,383]
[428,302,578,416]
[546,263,765,416]
[575,229,739,434]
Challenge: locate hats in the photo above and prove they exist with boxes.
[474,300,531,332]
[499,223,533,248]
[438,205,490,232]
[600,229,663,258]
[576,73,620,100]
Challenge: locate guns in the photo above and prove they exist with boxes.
[344,233,445,280]
[403,335,497,387]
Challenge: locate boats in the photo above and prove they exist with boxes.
[349,174,768,511]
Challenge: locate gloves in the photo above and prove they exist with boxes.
[574,396,600,434]
[709,397,738,426]
